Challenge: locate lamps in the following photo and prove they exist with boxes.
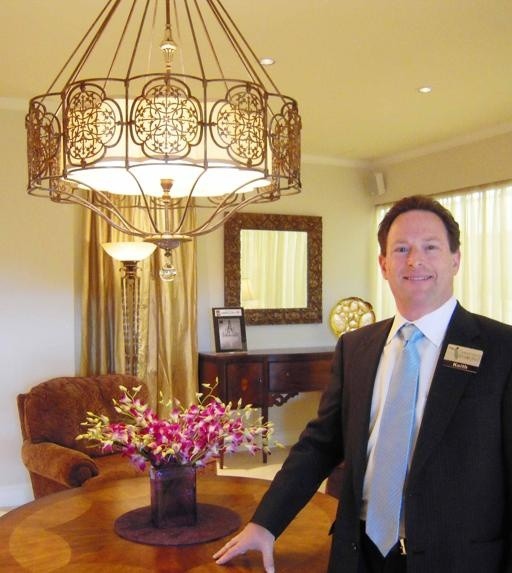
[25,0,300,281]
[101,242,157,377]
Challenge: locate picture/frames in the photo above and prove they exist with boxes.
[223,212,323,325]
[212,307,248,353]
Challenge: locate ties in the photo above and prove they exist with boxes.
[365,324,424,558]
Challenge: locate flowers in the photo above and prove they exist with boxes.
[74,377,286,476]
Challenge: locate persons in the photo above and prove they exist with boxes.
[212,195,511,572]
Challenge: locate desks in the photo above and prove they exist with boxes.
[198,346,336,469]
[0,477,342,573]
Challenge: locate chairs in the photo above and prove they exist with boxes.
[17,374,217,501]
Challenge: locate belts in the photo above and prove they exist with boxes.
[359,538,407,556]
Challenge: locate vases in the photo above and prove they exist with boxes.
[148,465,196,528]
